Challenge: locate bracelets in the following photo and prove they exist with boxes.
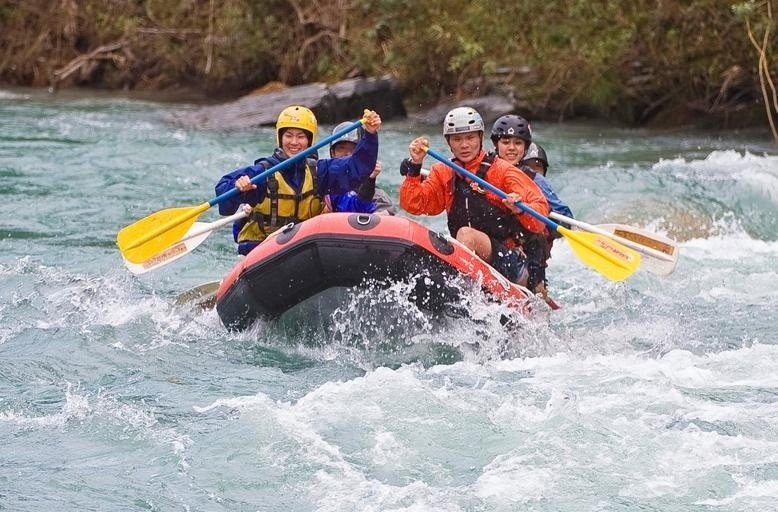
[510,210,525,217]
[399,158,422,177]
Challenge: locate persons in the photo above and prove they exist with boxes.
[399,106,549,288]
[233,149,383,244]
[215,106,382,257]
[330,121,396,217]
[490,114,573,295]
[521,141,549,177]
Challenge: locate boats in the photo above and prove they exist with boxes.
[214,212,532,338]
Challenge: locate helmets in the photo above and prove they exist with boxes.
[490,115,532,150]
[329,120,358,156]
[443,107,484,144]
[276,105,318,149]
[522,140,548,165]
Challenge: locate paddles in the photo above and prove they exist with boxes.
[120,211,248,275]
[419,140,639,283]
[417,168,679,276]
[177,211,400,311]
[117,108,374,263]
